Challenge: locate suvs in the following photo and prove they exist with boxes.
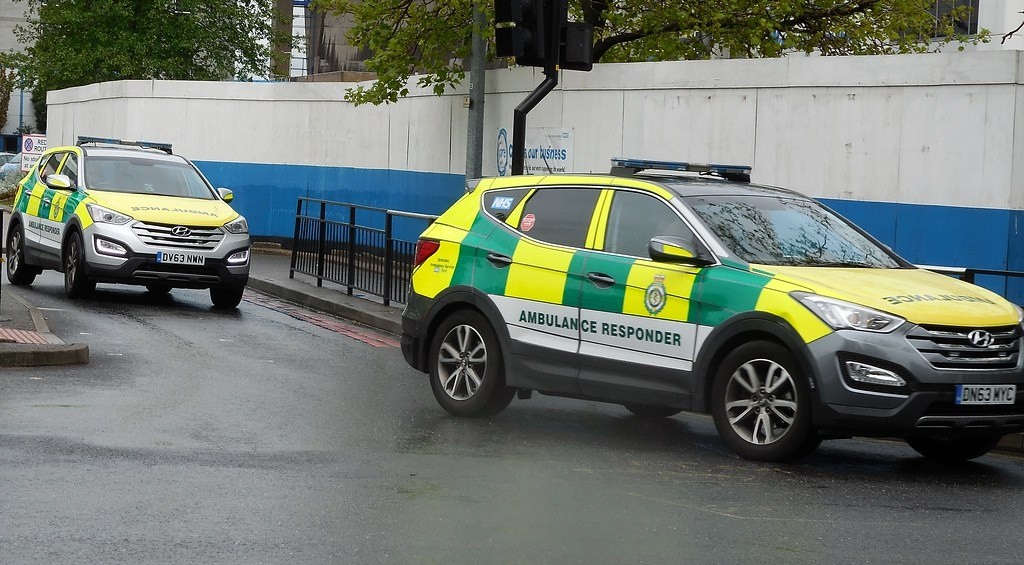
[400,157,1024,470]
[6,136,252,306]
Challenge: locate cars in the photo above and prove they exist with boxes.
[0,152,16,168]
[0,150,21,181]
